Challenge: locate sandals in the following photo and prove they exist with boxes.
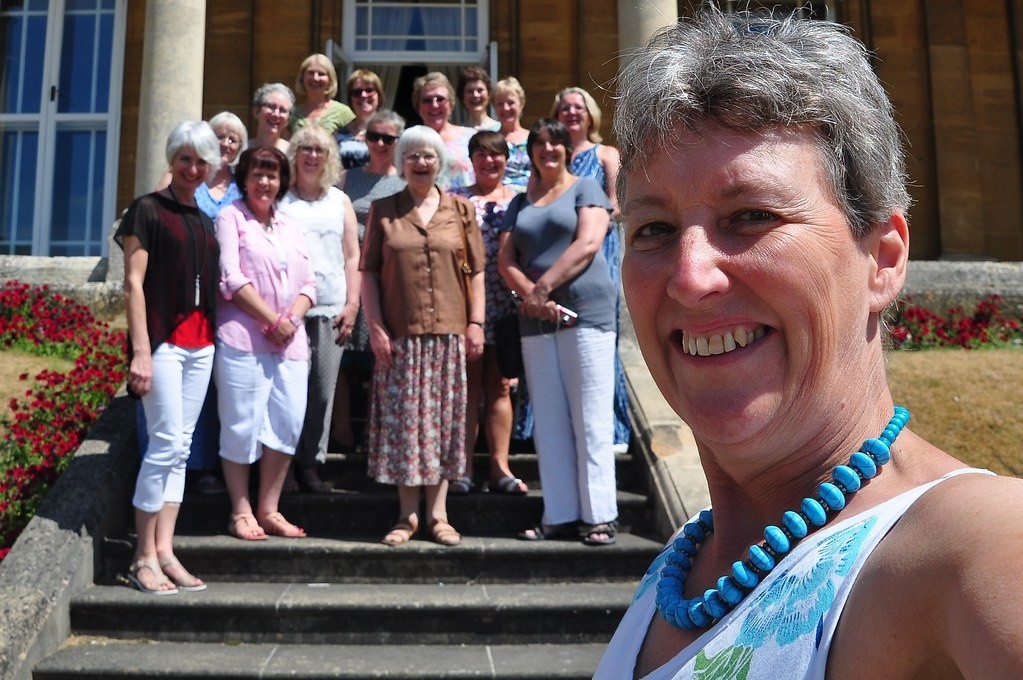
[257,513,307,537]
[282,478,302,494]
[426,519,461,546]
[447,476,473,495]
[228,513,268,541]
[305,473,333,494]
[582,520,618,545]
[383,520,416,546]
[494,477,530,496]
[518,526,580,541]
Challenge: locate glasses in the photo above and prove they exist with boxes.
[403,153,438,163]
[351,87,374,97]
[366,129,399,145]
[418,96,450,103]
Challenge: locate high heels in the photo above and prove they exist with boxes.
[157,556,207,592]
[126,556,179,595]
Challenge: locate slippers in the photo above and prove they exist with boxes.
[194,475,226,492]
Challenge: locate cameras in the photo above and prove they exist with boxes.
[557,305,578,327]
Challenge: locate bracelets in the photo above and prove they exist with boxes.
[269,314,282,334]
[287,312,303,328]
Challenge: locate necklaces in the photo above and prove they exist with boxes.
[655,404,912,631]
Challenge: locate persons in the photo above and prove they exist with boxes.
[111,53,622,592]
[591,0,1023,680]
